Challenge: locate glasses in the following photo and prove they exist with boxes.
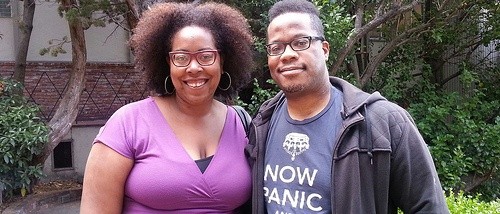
[263,36,325,57]
[168,50,222,67]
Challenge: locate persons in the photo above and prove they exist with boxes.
[79,1,256,214]
[243,0,451,214]
